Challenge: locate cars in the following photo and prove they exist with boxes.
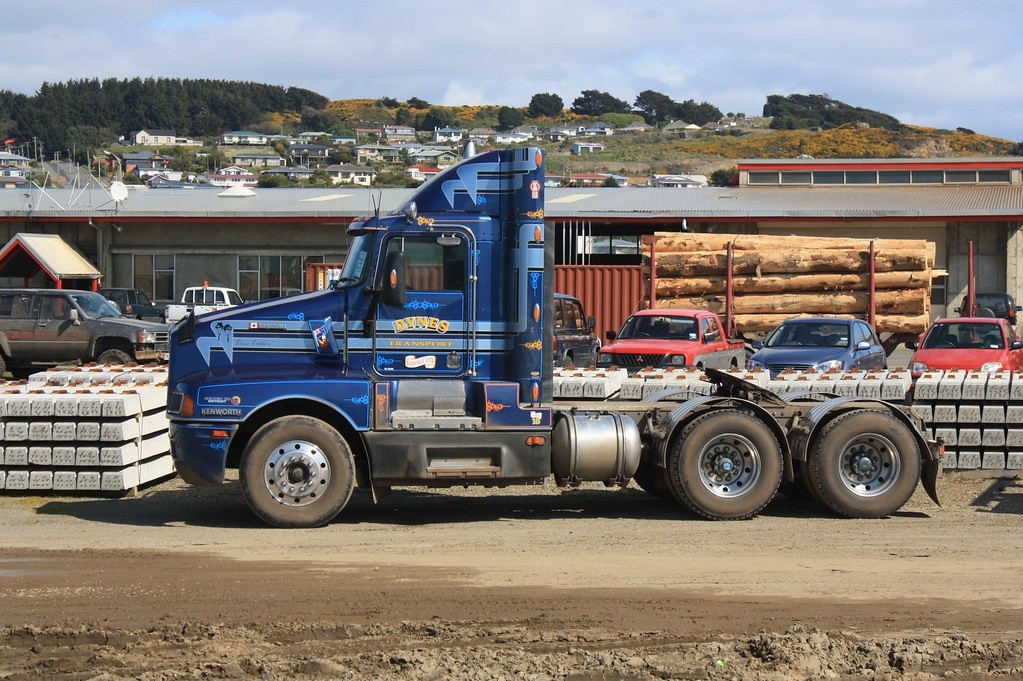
[87,288,165,324]
[0,289,170,381]
[905,317,1023,380]
[552,293,601,368]
[954,292,1022,324]
[746,318,887,380]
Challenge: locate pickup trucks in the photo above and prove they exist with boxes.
[165,287,244,324]
[245,287,303,304]
[595,308,746,374]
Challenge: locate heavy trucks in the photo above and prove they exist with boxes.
[165,140,945,529]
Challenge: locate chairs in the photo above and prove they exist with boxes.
[653,321,693,338]
[807,332,847,346]
[61,301,77,319]
[980,334,1001,349]
[943,334,959,348]
